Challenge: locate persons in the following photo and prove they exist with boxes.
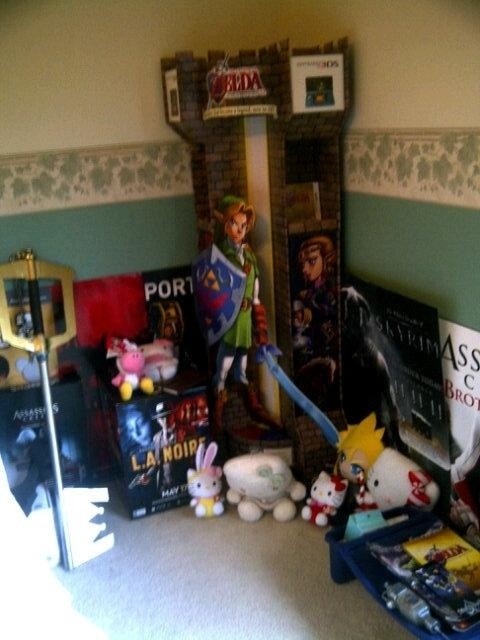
[141,402,178,488]
[125,409,152,447]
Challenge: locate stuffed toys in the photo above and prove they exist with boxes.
[223,449,306,522]
[186,442,226,518]
[300,471,349,527]
[106,335,154,401]
[138,339,180,382]
[334,412,387,485]
[366,449,440,512]
